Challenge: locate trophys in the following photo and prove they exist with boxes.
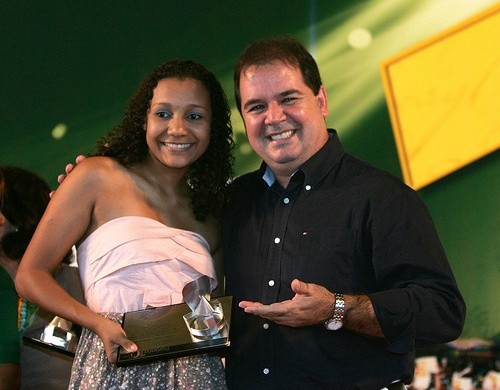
[115,276,233,363]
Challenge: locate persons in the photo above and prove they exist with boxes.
[0,167,87,390]
[14,61,236,390]
[48,36,467,390]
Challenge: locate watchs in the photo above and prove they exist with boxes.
[326,293,345,331]
